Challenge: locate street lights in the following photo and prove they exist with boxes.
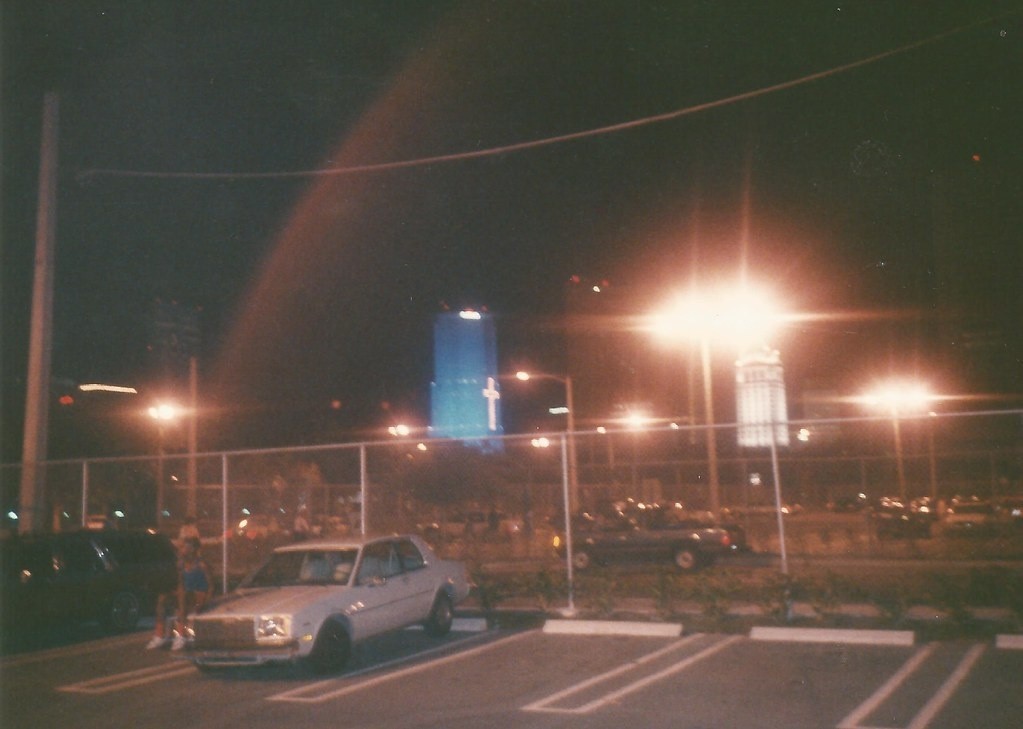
[530,433,578,615]
[151,404,177,530]
[518,370,582,511]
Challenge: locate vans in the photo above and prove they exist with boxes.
[884,510,1023,618]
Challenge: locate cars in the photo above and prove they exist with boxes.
[195,532,473,674]
[0,525,176,652]
[552,509,746,575]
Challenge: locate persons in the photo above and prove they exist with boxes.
[178,515,202,556]
[146,537,217,652]
[292,504,310,541]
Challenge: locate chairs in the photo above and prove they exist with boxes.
[335,563,353,581]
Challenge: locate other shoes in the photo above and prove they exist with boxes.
[171,636,186,650]
[147,636,164,649]
